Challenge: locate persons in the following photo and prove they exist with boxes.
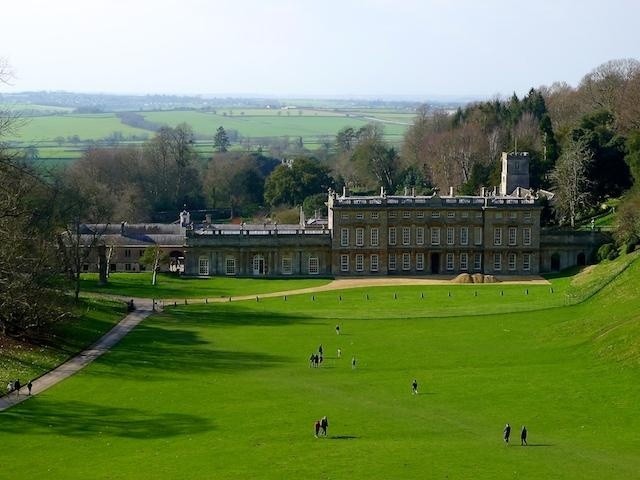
[27,380,32,395]
[308,343,324,369]
[14,378,21,397]
[320,415,328,437]
[7,380,15,393]
[314,420,320,438]
[336,324,340,335]
[503,423,510,443]
[411,379,418,395]
[520,426,527,446]
[337,348,341,358]
